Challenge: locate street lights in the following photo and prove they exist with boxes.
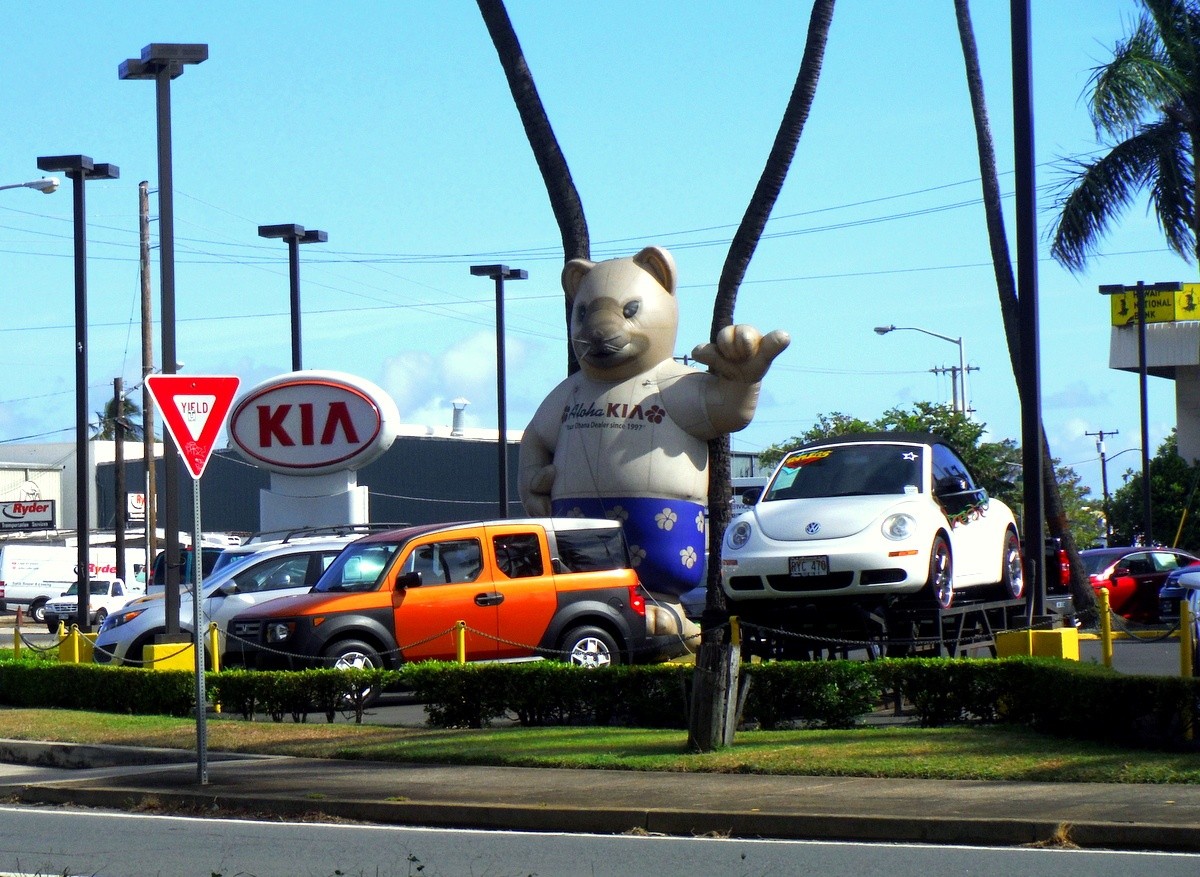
[114,357,184,580]
[875,325,970,432]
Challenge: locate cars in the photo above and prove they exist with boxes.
[1057,545,1195,624]
[720,430,1028,608]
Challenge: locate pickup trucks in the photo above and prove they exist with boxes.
[48,576,137,633]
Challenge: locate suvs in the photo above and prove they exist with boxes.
[92,518,425,669]
[238,516,650,672]
